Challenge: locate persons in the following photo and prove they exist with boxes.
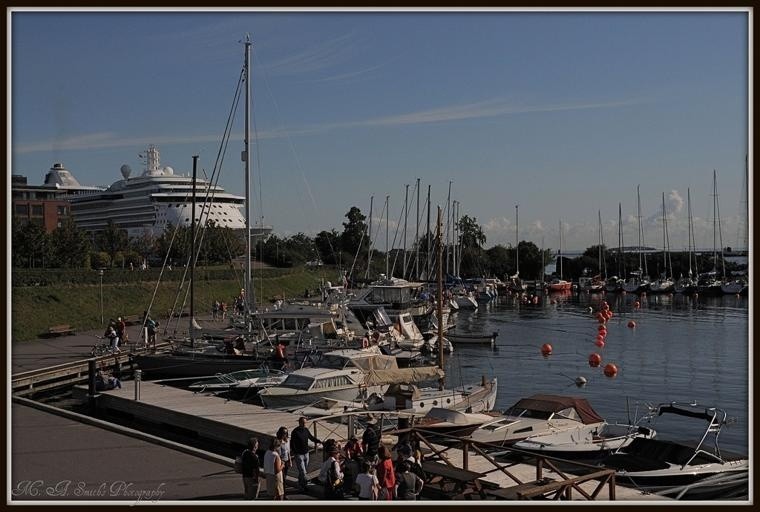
[211,290,245,323]
[117,316,127,348]
[371,329,380,344]
[415,283,478,304]
[274,426,292,495]
[240,436,265,500]
[263,437,286,500]
[291,416,324,489]
[143,310,161,351]
[318,415,427,501]
[108,326,121,356]
[128,262,188,272]
[105,318,119,349]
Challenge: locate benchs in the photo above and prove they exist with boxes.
[468,479,499,490]
[121,314,142,324]
[47,323,76,339]
[180,304,198,317]
[422,484,462,497]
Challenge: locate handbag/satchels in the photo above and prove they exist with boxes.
[328,460,344,488]
[380,488,389,500]
[371,475,379,500]
[235,449,249,474]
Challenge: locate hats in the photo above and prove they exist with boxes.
[363,415,377,424]
[367,450,377,455]
[350,434,359,441]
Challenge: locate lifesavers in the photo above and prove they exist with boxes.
[361,338,368,348]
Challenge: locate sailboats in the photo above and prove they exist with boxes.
[128,32,748,377]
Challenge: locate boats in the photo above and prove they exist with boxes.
[192,368,748,503]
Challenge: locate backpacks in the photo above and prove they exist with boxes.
[403,458,426,482]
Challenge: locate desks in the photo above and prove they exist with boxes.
[423,460,486,500]
[485,476,560,500]
